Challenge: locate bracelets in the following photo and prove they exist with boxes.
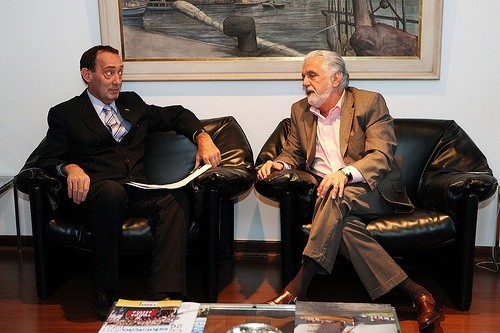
[195,129,207,141]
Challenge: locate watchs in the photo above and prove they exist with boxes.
[338,168,352,184]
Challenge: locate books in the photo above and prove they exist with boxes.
[98,298,210,333]
[294,301,402,333]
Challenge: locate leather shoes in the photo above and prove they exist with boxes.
[95,287,116,320]
[414,293,443,329]
[259,289,298,305]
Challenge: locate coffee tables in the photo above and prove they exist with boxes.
[204,303,295,333]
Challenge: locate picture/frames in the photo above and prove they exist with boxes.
[99,0,444,80]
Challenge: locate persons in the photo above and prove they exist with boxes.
[37,46,221,319]
[256,50,441,332]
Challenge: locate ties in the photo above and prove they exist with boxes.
[104,105,126,141]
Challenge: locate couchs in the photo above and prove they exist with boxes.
[253,118,498,311]
[12,116,254,303]
